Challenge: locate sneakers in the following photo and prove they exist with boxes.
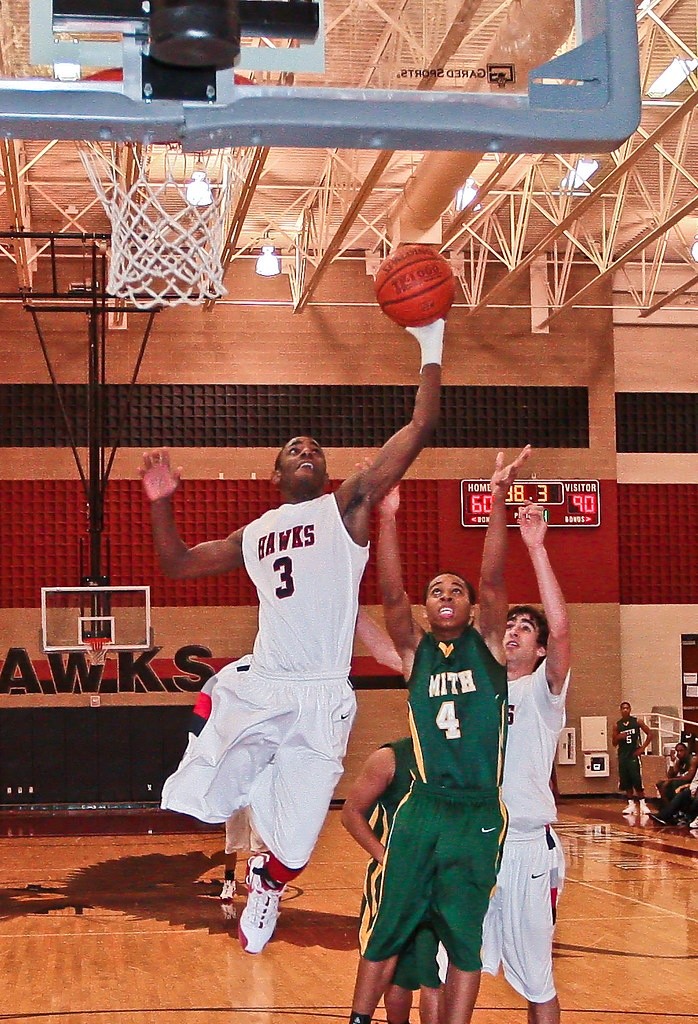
[238,856,287,955]
[622,804,636,814]
[221,904,236,921]
[640,804,651,814]
[220,879,237,899]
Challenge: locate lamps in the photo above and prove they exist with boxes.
[645,57,698,98]
[256,246,280,277]
[561,156,600,191]
[187,172,214,205]
[453,179,481,212]
[54,63,80,83]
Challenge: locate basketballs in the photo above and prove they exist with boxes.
[371,244,457,328]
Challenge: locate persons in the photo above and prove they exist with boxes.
[611,701,652,815]
[341,738,441,1024]
[351,444,533,1024]
[138,319,451,956]
[422,507,575,1024]
[652,743,698,830]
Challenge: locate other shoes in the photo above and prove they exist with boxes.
[690,816,698,828]
[652,814,669,825]
